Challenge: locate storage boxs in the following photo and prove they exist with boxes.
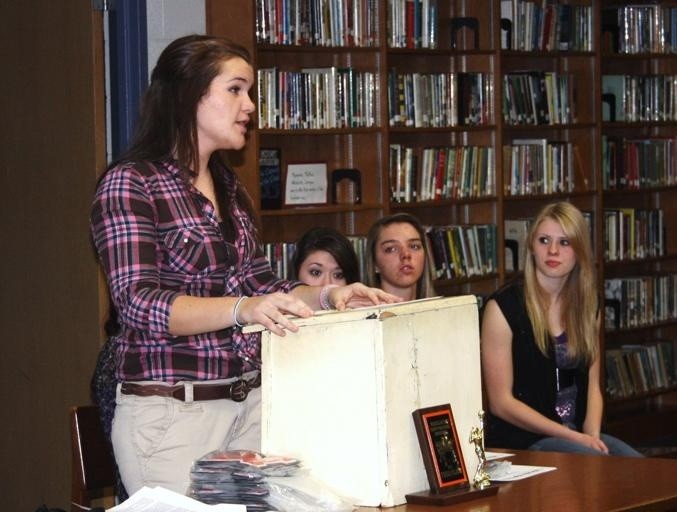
[238,287,492,508]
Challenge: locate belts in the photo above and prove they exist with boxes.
[121,372,261,402]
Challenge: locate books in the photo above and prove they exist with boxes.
[255,0,677,400]
[413,403,469,492]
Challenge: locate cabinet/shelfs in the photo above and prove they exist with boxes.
[206,2,676,449]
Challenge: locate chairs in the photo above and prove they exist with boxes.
[68,404,124,511]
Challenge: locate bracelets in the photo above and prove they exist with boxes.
[231,295,248,327]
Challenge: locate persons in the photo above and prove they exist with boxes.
[479,200,644,458]
[87,33,405,500]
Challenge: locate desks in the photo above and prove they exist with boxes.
[73,448,669,511]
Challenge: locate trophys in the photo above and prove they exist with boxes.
[469,409,493,487]
